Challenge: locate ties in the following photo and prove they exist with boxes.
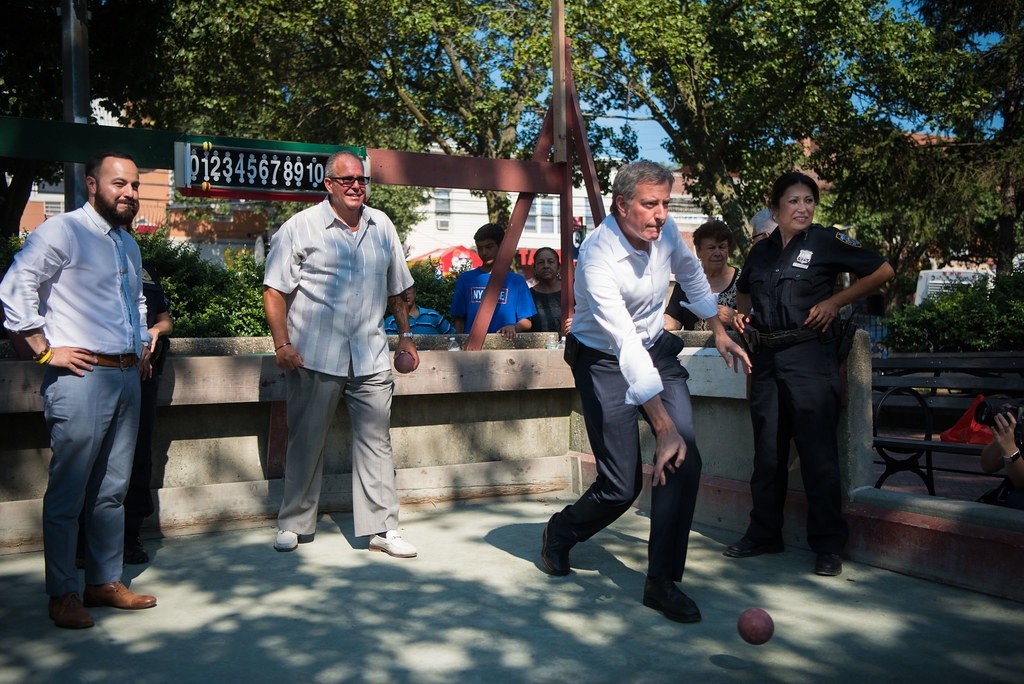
[109,229,142,360]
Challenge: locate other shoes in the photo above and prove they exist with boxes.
[124,534,150,565]
[275,528,299,551]
[368,529,418,558]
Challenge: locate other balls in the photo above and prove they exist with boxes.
[738,608,774,645]
[394,354,415,374]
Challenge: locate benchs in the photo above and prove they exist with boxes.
[871,353,1024,509]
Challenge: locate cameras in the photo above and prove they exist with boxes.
[973,400,1024,446]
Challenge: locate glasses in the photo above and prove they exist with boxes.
[329,176,371,186]
[748,231,764,244]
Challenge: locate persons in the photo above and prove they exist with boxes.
[980,412,1024,510]
[723,172,895,576]
[450,223,537,341]
[262,151,420,559]
[75,258,173,569]
[979,258,997,272]
[664,220,742,331]
[0,151,157,628]
[529,248,576,334]
[750,210,778,246]
[384,283,456,336]
[541,160,752,624]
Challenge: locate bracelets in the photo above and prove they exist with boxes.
[275,343,291,352]
[33,339,54,366]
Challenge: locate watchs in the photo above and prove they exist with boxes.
[1002,448,1020,463]
[399,332,413,339]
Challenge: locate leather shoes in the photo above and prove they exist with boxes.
[541,524,571,576]
[814,553,842,576]
[48,592,95,629]
[642,577,702,623]
[92,581,157,609]
[726,536,785,558]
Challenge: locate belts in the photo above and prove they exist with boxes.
[760,325,819,349]
[83,351,139,368]
